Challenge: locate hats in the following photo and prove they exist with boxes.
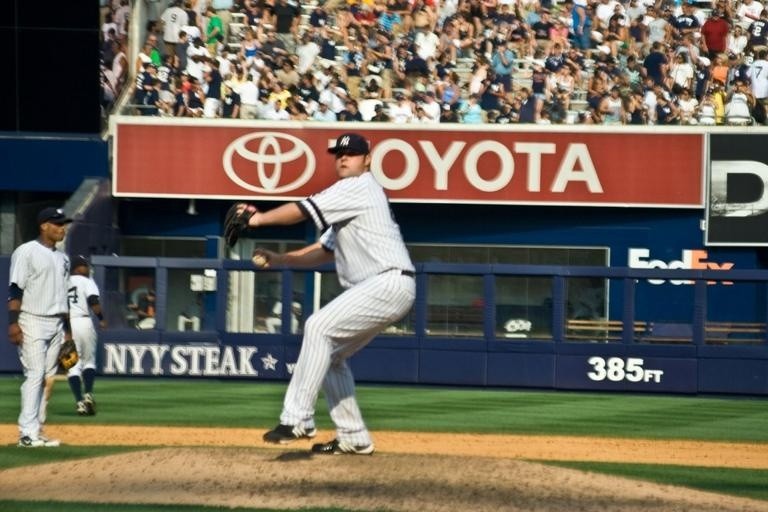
[36,206,74,227]
[132,0,768,111]
[327,132,371,156]
[69,254,91,269]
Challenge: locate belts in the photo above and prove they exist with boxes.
[386,268,416,276]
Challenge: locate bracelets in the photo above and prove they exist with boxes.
[62,317,71,335]
[8,310,18,325]
[96,312,104,321]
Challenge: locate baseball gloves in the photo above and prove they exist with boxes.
[59,340,80,370]
[222,204,257,248]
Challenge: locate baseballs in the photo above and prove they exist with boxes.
[252,252,268,268]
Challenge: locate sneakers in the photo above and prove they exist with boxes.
[262,423,317,445]
[16,435,62,450]
[75,392,98,416]
[311,438,376,457]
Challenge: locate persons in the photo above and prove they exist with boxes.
[265,292,302,334]
[60,254,107,416]
[236,134,416,456]
[128,290,156,331]
[7,207,72,447]
[567,276,603,341]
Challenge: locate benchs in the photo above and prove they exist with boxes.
[450,57,596,112]
[565,320,768,345]
[403,305,502,337]
[227,3,350,62]
